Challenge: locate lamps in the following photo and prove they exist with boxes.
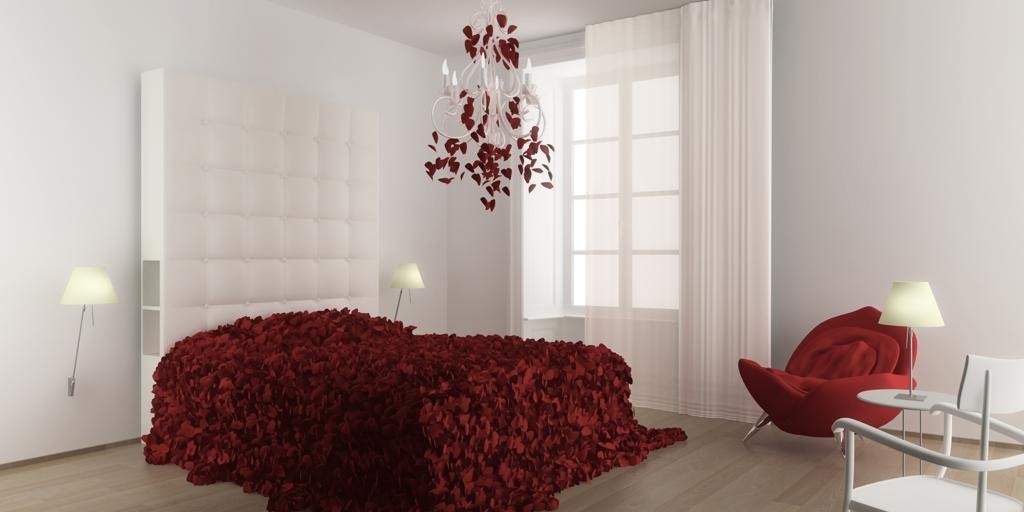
[389,260,428,319]
[875,278,946,401]
[59,263,121,396]
[415,8,559,205]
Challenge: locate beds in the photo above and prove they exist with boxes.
[140,308,689,510]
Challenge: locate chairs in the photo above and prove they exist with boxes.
[737,294,918,453]
[832,355,1024,511]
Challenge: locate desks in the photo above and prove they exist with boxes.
[858,388,955,484]
